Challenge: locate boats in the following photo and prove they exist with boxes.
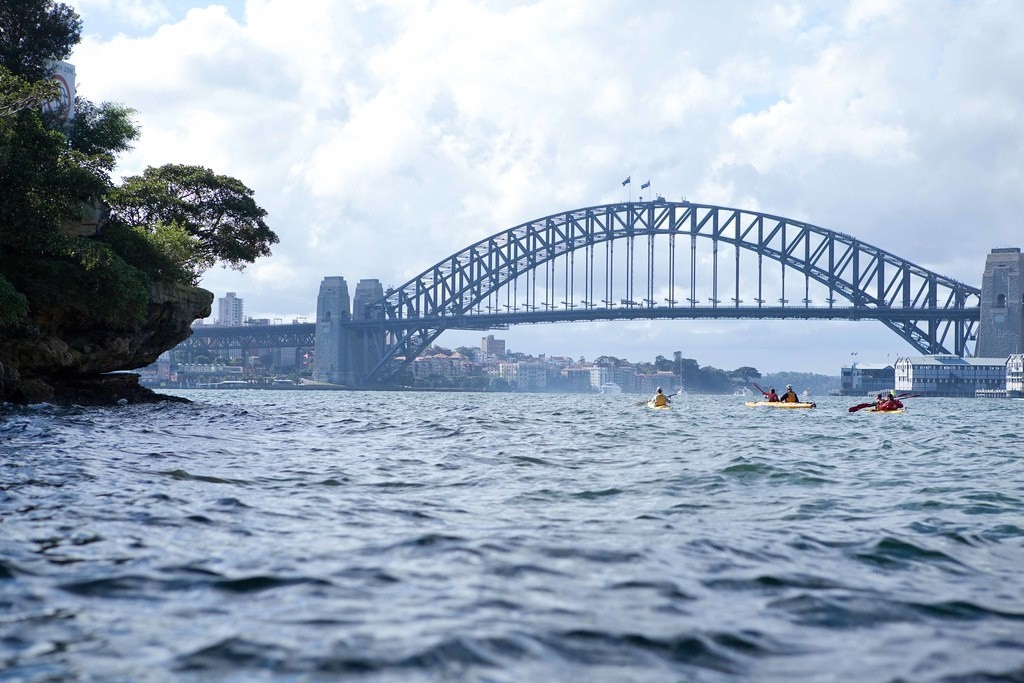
[647,399,674,410]
[745,400,817,409]
[868,407,905,414]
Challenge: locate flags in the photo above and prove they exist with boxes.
[622,176,650,189]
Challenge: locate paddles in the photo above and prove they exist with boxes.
[848,391,922,413]
[631,390,687,408]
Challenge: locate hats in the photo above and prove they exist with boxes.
[785,384,792,389]
[657,387,663,391]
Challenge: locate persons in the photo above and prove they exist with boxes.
[875,393,904,411]
[651,387,672,407]
[762,383,799,403]
